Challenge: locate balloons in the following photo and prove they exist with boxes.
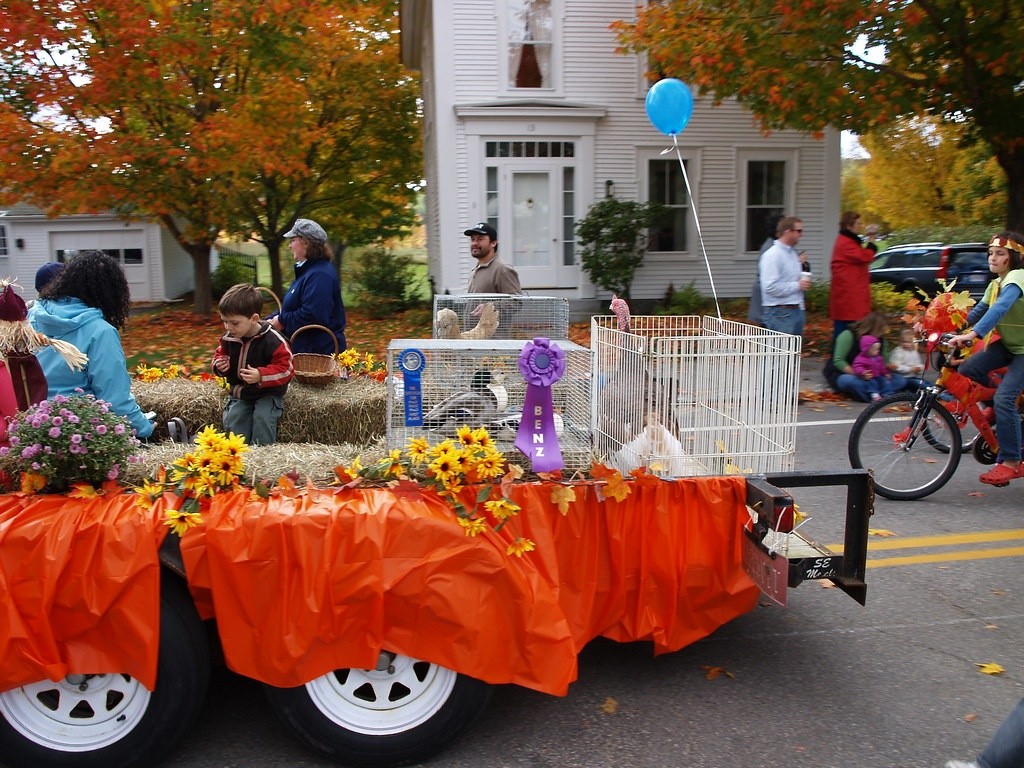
[643,76,694,137]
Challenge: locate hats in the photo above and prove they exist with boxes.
[35,262,66,290]
[283,219,327,244]
[464,223,499,240]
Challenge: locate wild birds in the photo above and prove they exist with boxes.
[437,300,501,340]
[420,366,563,442]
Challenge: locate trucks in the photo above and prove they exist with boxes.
[0,378,876,768]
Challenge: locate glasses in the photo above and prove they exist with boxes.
[789,228,803,234]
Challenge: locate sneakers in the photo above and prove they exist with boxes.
[872,397,882,404]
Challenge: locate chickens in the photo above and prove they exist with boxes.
[599,293,683,477]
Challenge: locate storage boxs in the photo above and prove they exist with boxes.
[433,293,571,340]
[386,338,595,472]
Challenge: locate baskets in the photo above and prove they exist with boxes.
[289,325,338,387]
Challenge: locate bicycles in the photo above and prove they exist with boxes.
[848,328,1024,501]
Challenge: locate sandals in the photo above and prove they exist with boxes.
[980,407,995,421]
[979,461,1024,485]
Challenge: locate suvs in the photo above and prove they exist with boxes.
[866,242,1000,309]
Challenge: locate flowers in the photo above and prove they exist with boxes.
[330,346,389,383]
[0,386,140,483]
[329,423,534,558]
[133,423,251,538]
[134,363,231,392]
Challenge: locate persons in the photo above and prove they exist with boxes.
[0,341,21,490]
[946,231,1024,485]
[827,210,878,357]
[209,282,295,445]
[821,310,926,404]
[747,214,812,406]
[264,218,348,380]
[24,248,159,447]
[463,222,523,339]
[942,697,1024,768]
[0,274,89,414]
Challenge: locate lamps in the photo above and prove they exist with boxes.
[605,179,615,197]
[14,238,24,248]
